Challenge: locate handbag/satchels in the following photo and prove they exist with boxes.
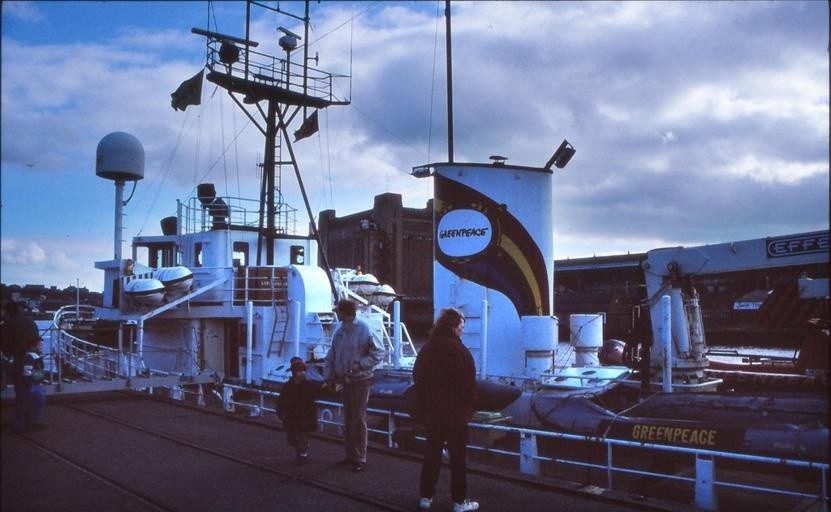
[0,356,14,385]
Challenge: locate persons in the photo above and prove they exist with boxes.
[0,298,44,433]
[413,308,481,511]
[276,357,328,460]
[324,300,382,472]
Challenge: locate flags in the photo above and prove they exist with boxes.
[294,110,319,143]
[171,70,205,111]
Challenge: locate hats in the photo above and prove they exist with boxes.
[290,357,306,372]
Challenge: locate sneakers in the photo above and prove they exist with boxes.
[419,496,480,512]
[339,458,366,472]
[297,451,312,466]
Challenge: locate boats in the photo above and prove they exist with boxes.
[41,0,831,510]
